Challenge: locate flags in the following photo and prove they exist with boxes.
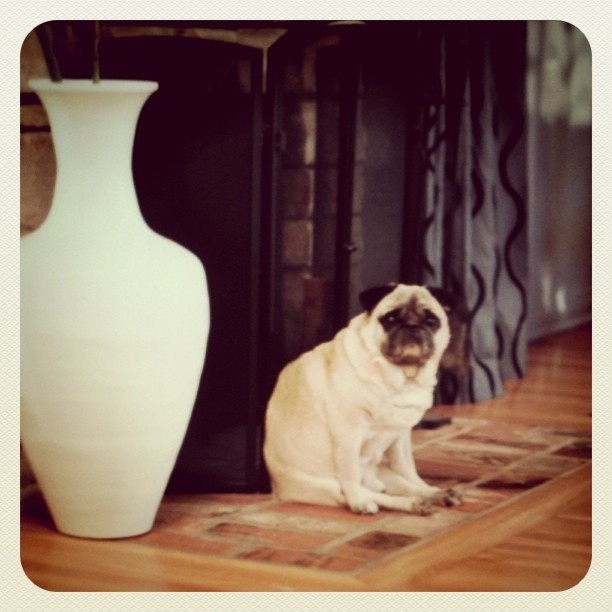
[21,79,212,540]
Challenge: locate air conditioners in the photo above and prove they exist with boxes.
[262,279,465,518]
